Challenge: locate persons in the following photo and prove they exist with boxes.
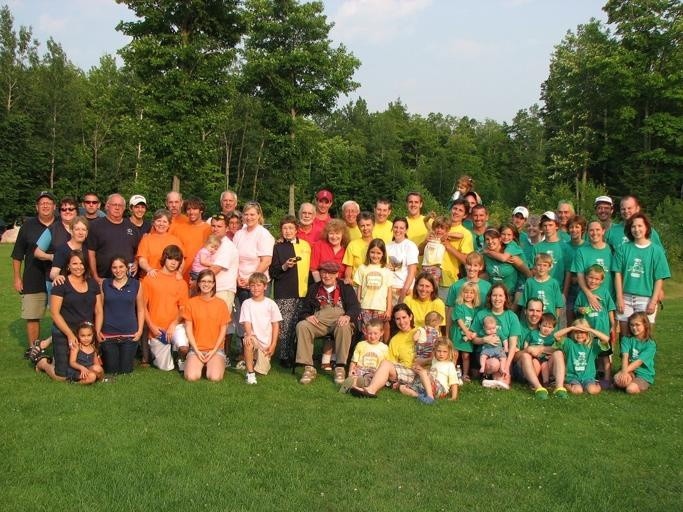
[446,192,670,399]
[457,178,482,205]
[13,190,282,383]
[269,191,473,404]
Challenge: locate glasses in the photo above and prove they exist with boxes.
[212,215,224,219]
[61,208,76,211]
[85,201,98,204]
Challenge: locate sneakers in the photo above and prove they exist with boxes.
[23,339,258,385]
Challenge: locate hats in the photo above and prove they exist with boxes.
[36,191,54,201]
[317,262,339,271]
[513,206,529,218]
[317,191,332,200]
[540,211,558,221]
[129,195,147,206]
[594,196,612,206]
